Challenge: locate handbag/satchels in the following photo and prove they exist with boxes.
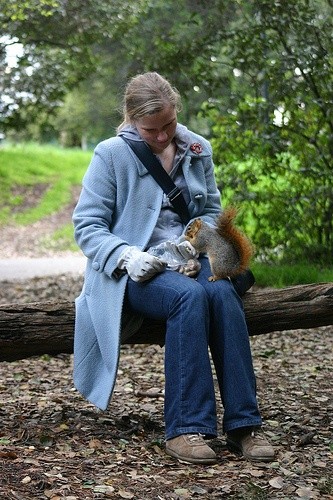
[231,265,255,295]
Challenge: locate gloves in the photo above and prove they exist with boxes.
[166,239,199,261]
[116,246,164,283]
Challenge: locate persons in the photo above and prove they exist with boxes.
[72,71,275,464]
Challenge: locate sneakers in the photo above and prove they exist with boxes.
[226,426,275,461]
[166,434,218,465]
